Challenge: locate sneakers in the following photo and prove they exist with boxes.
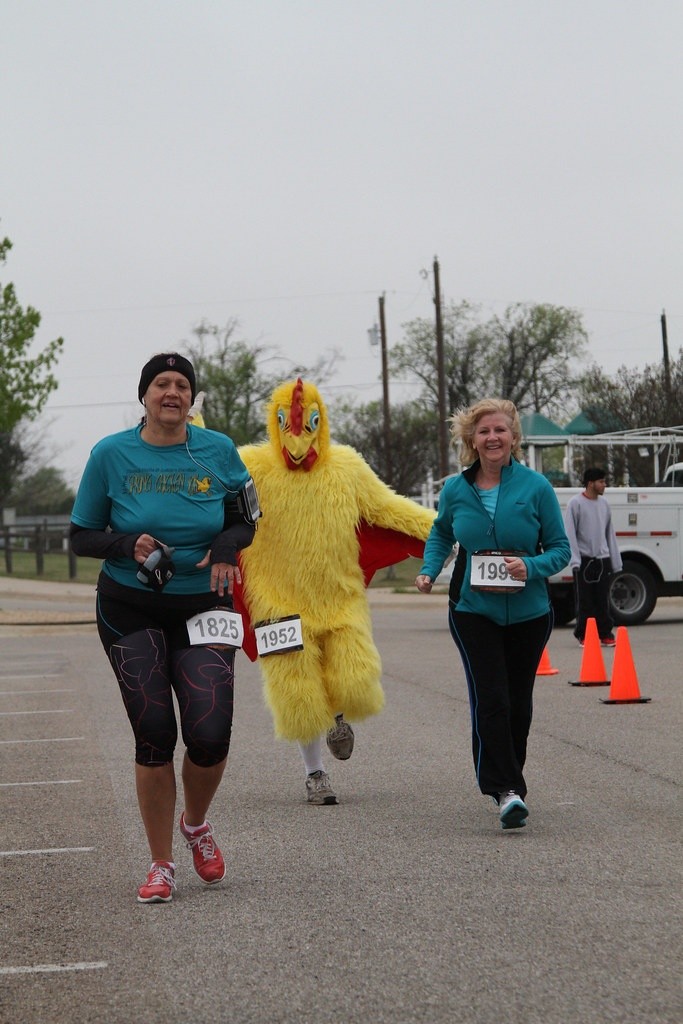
[178,811,226,884]
[602,639,615,646]
[326,714,355,761]
[137,861,177,902]
[496,790,529,829]
[579,639,584,647]
[304,770,337,804]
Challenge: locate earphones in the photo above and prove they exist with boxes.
[141,397,145,405]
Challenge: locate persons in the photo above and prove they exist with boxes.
[566,468,626,648]
[414,396,572,830]
[188,379,462,807]
[64,353,261,909]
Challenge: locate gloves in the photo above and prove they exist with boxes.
[443,545,458,568]
[572,567,579,576]
[615,571,623,581]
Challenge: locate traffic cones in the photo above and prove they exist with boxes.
[568,618,611,687]
[598,626,651,704]
[536,645,559,675]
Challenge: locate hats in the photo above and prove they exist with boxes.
[583,468,605,486]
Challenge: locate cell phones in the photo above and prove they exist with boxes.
[242,476,261,521]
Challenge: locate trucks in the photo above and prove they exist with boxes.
[458,426,683,624]
